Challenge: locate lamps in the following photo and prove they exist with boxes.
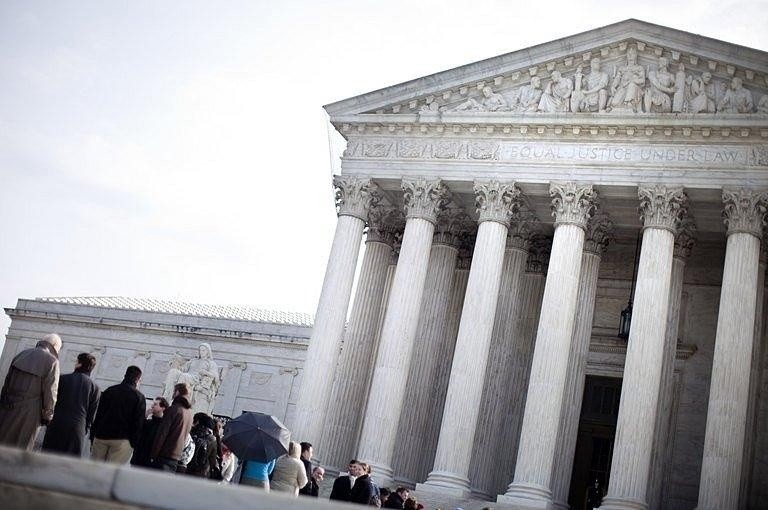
[617,229,640,344]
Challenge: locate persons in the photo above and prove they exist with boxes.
[435,508,441,510]
[163,342,219,409]
[481,506,492,510]
[176,409,424,510]
[1,332,63,450]
[41,352,101,460]
[132,397,170,469]
[439,40,768,114]
[150,382,195,474]
[88,365,146,469]
